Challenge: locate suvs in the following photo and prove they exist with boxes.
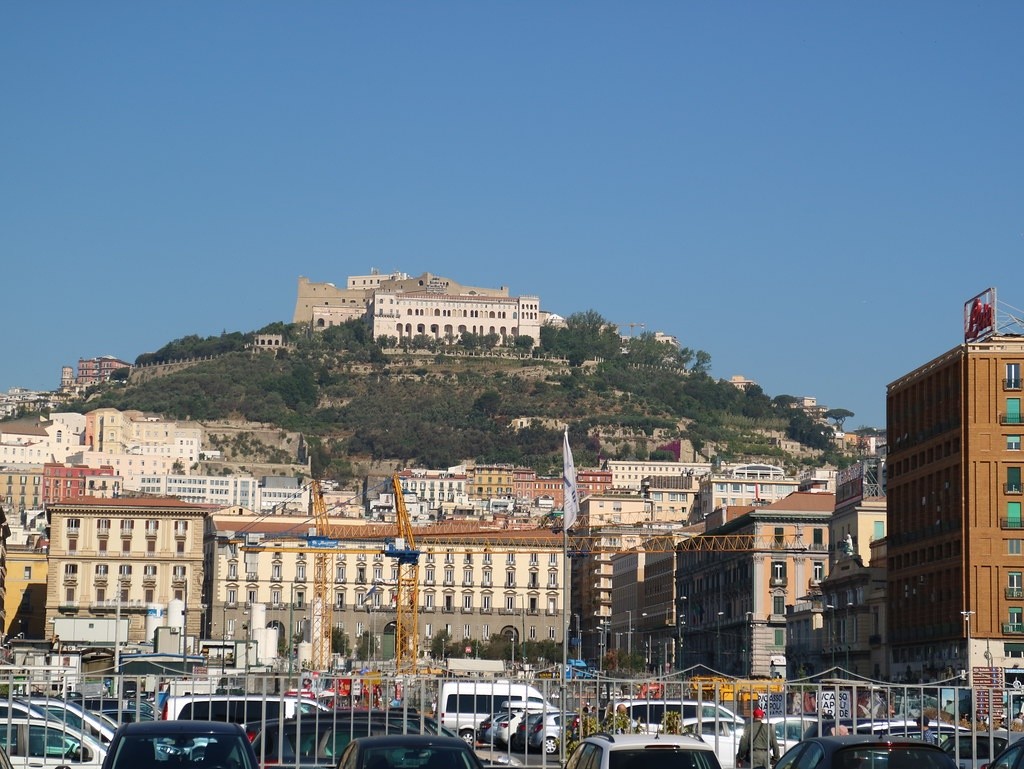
[563,732,723,769]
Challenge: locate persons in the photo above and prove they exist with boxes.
[913,716,935,744]
[569,704,641,741]
[431,699,437,715]
[831,725,849,736]
[736,708,779,769]
[962,712,1024,731]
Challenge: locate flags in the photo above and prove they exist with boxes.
[563,431,580,532]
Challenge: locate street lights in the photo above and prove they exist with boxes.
[199,595,692,679]
[961,611,976,687]
[745,612,754,679]
[718,612,724,673]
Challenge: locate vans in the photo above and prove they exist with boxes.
[604,699,745,735]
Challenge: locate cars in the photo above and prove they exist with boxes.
[761,715,819,769]
[683,717,746,769]
[988,737,1024,769]
[0,672,579,769]
[771,730,958,769]
[799,711,971,749]
[938,731,1024,769]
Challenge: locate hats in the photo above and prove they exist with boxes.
[913,716,929,727]
[753,709,764,719]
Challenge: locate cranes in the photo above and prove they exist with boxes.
[239,474,806,677]
[217,478,688,673]
